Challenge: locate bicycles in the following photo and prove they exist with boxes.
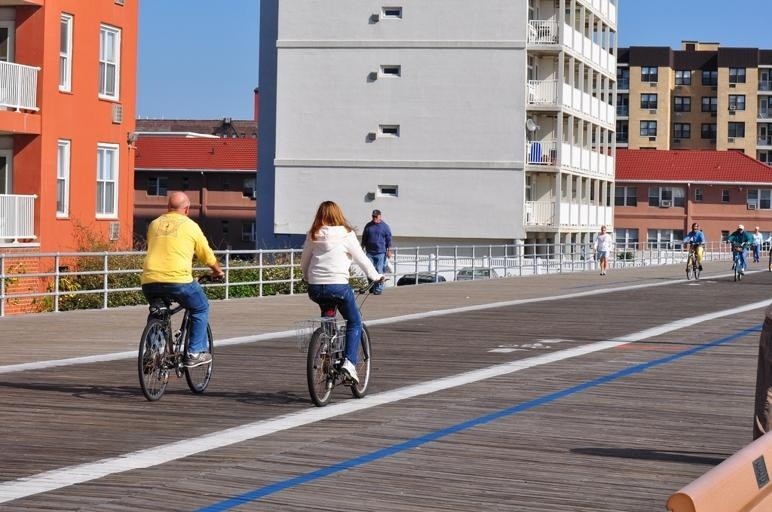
[307,275,390,406]
[683,241,701,280]
[726,241,747,282]
[138,273,226,402]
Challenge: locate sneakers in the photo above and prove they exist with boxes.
[340,356,361,386]
[141,348,155,375]
[368,287,382,296]
[183,351,214,369]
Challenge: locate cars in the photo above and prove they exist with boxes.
[398,266,498,286]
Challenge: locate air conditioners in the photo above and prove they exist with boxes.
[661,200,672,208]
[748,204,756,210]
[730,104,737,110]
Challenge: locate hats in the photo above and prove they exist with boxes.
[737,225,744,229]
[372,209,380,217]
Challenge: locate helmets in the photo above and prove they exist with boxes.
[692,223,698,227]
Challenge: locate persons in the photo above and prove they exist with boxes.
[592,225,612,275]
[752,226,764,263]
[360,209,394,296]
[301,200,382,385]
[683,222,704,273]
[752,303,771,442]
[140,191,227,374]
[725,224,753,277]
[763,236,772,272]
